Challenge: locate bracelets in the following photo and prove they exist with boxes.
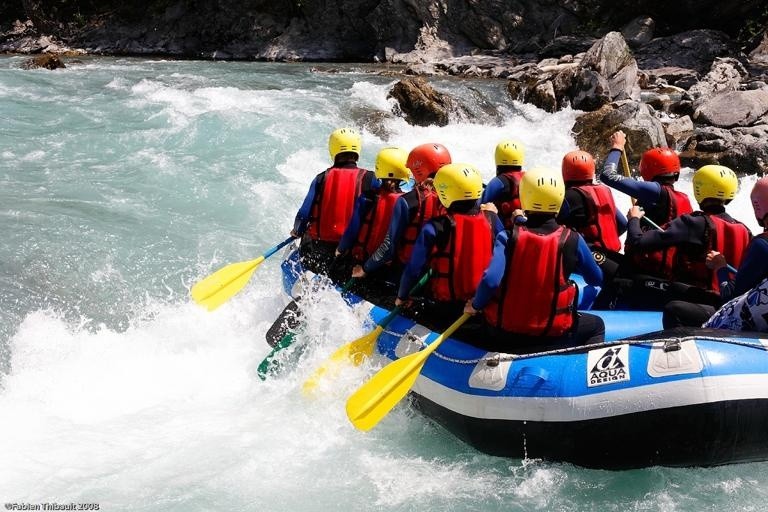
[609,147,624,155]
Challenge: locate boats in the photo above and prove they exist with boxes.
[278,220,764,463]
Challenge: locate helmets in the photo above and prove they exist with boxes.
[329,127,362,160]
[639,148,768,220]
[374,141,595,214]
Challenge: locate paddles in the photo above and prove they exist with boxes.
[191,235,295,316]
[266,255,341,348]
[345,312,472,433]
[304,267,432,397]
[258,277,356,381]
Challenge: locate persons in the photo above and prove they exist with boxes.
[393,161,505,329]
[288,126,377,274]
[461,167,607,350]
[328,145,415,282]
[660,174,768,328]
[476,140,527,230]
[554,149,627,254]
[348,143,452,294]
[589,126,695,282]
[598,164,753,310]
